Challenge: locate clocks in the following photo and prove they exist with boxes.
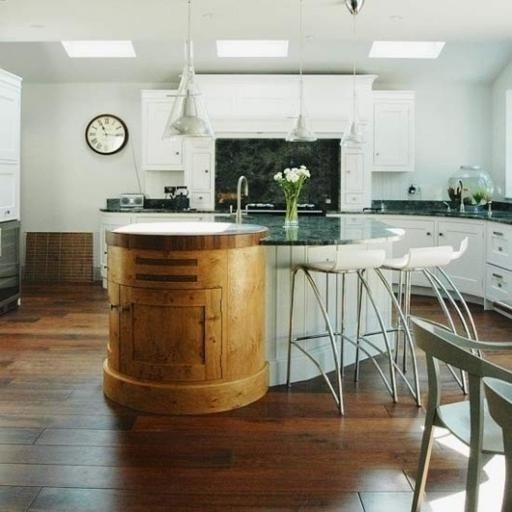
[85,113,129,156]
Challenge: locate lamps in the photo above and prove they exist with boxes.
[161,1,214,141]
[285,2,318,142]
[340,1,370,149]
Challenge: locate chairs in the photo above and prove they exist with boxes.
[406,314,512,512]
[481,376,511,511]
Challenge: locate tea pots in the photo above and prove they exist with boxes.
[170,186,189,210]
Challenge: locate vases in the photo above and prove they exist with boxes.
[285,199,299,227]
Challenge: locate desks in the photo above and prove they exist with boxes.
[103,215,406,417]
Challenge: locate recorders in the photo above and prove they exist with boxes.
[119,192,145,208]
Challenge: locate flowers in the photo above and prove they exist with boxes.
[273,164,311,198]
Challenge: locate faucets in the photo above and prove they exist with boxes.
[457,178,465,213]
[229,175,250,222]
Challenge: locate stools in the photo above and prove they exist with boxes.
[286,250,424,416]
[383,235,488,396]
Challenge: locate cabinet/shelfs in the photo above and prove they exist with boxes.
[371,89,415,173]
[184,74,376,212]
[377,202,512,319]
[142,89,185,172]
[0,69,24,222]
